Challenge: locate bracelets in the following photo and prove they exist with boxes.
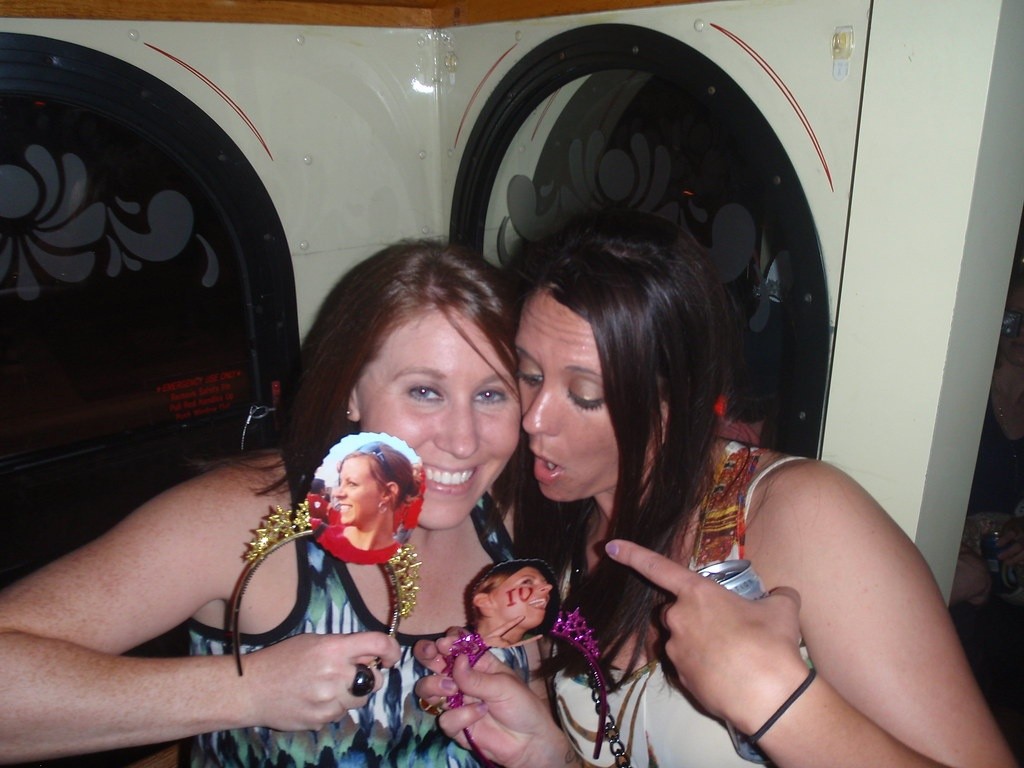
[747,669,818,746]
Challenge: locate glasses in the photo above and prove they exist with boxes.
[357,443,397,483]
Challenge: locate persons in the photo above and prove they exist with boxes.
[319,443,422,566]
[462,559,554,648]
[413,227,1018,768]
[0,237,527,768]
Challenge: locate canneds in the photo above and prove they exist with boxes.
[694,559,788,765]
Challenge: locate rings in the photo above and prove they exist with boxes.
[417,692,446,716]
[349,664,376,698]
